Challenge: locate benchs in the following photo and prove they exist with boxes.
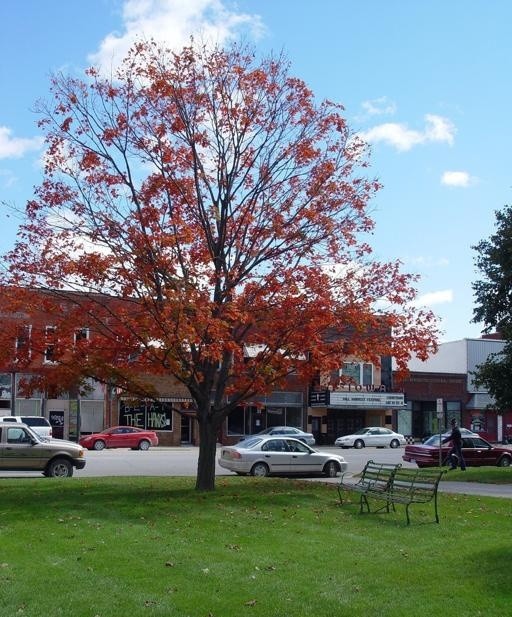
[338,460,447,526]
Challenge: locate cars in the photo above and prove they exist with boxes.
[0,423,86,477]
[80,426,158,452]
[402,428,512,468]
[335,427,406,449]
[220,424,348,478]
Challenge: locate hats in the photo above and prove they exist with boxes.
[448,418,456,425]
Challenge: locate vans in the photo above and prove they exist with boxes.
[0,415,52,438]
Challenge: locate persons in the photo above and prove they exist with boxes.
[442,419,468,471]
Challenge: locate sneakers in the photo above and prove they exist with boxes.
[448,466,466,473]
